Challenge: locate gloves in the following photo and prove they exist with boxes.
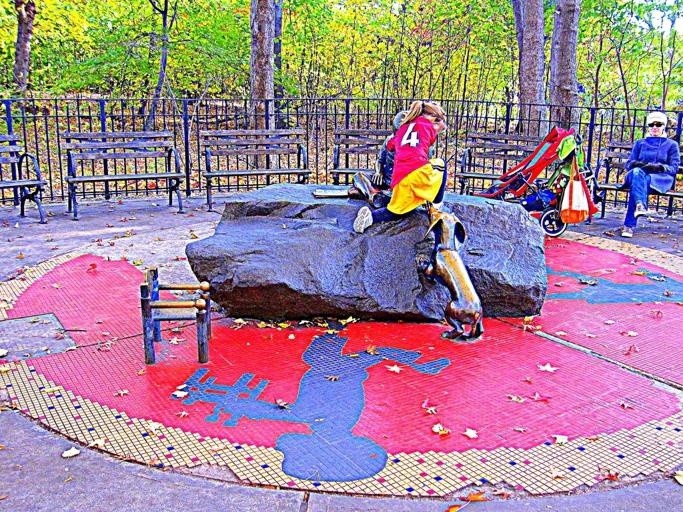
[644,162,664,172]
[632,161,643,168]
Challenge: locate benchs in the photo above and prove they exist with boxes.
[59,130,187,221]
[0,133,47,225]
[585,142,683,227]
[326,127,393,186]
[197,127,314,214]
[453,132,550,198]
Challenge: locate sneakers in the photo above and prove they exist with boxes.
[431,201,450,213]
[353,206,372,233]
[633,203,647,217]
[621,225,633,237]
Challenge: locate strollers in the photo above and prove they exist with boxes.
[466,125,606,238]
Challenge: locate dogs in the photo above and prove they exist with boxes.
[414,206,485,342]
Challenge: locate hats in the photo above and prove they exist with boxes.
[647,111,668,126]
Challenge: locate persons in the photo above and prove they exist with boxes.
[352,110,436,209]
[352,100,452,235]
[616,110,681,239]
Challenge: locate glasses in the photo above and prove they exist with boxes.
[648,123,662,127]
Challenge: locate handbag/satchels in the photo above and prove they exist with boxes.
[561,179,589,223]
